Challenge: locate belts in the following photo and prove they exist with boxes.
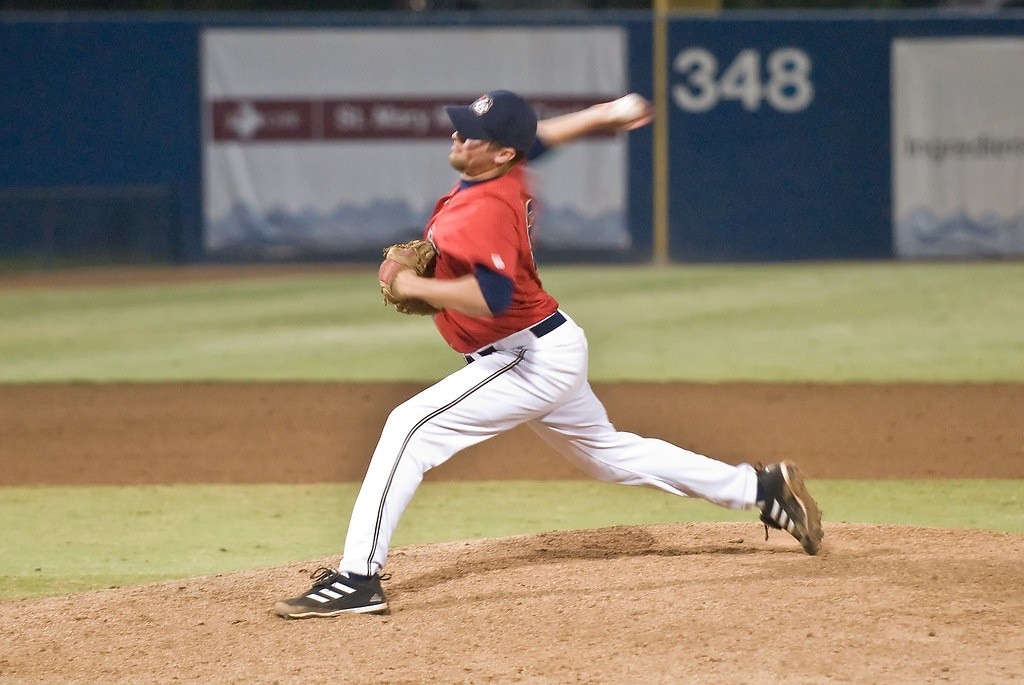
[463,312,567,365]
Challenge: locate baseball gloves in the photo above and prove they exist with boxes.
[377,239,446,317]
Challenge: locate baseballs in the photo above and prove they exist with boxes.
[615,91,647,119]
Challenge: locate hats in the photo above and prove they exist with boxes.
[443,90,537,155]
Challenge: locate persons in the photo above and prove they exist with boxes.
[272,90,825,618]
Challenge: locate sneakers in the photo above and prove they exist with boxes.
[754,459,825,556]
[275,567,392,618]
